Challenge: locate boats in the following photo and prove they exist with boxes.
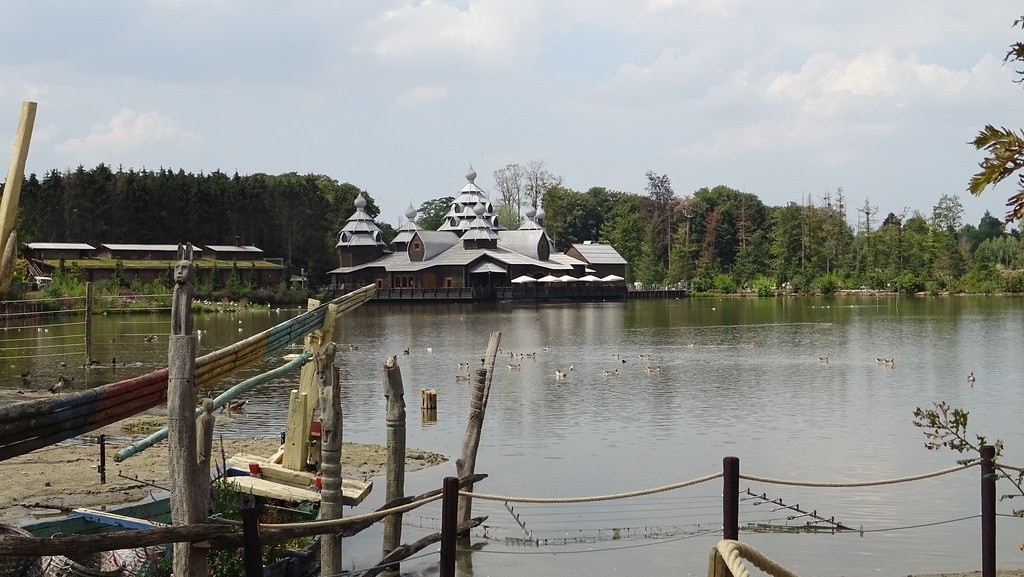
[1,284,373,576]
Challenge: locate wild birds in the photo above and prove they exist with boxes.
[12,295,980,395]
